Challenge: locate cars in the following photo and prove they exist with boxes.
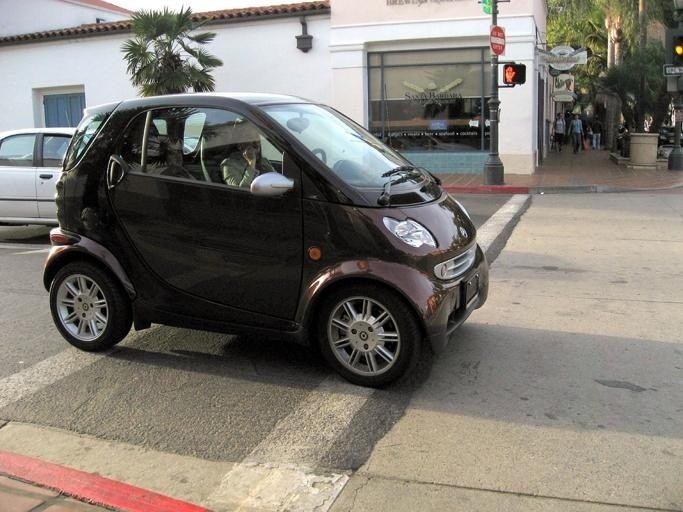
[43,91,490,388]
[380,131,477,152]
[660,124,683,147]
[0,127,75,227]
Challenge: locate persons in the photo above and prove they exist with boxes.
[219,119,276,188]
[552,113,603,154]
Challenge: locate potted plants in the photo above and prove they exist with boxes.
[602,46,666,165]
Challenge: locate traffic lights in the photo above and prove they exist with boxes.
[674,34,683,65]
[505,64,518,84]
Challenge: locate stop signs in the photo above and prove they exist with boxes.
[490,24,506,55]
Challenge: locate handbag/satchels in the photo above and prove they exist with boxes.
[582,135,588,150]
